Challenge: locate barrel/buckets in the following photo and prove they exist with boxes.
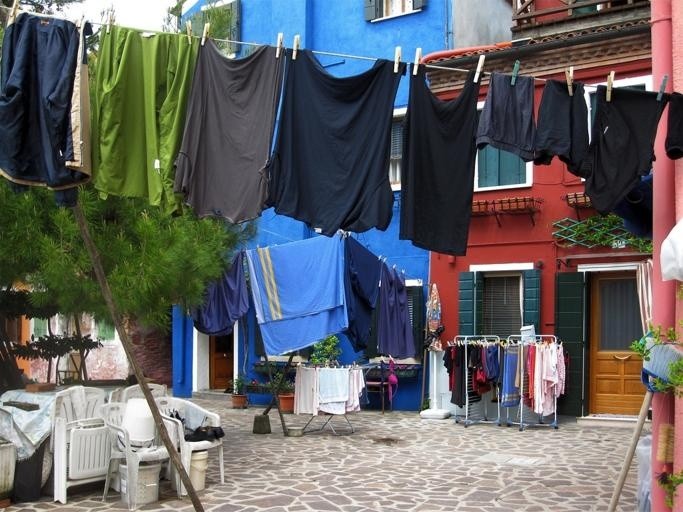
[118,460,160,507]
[170,449,208,493]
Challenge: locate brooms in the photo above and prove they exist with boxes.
[604,337,683,512]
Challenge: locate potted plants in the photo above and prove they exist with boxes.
[227,375,250,409]
[270,373,293,413]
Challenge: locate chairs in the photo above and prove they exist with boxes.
[96,402,180,511]
[153,396,224,496]
[49,385,119,505]
